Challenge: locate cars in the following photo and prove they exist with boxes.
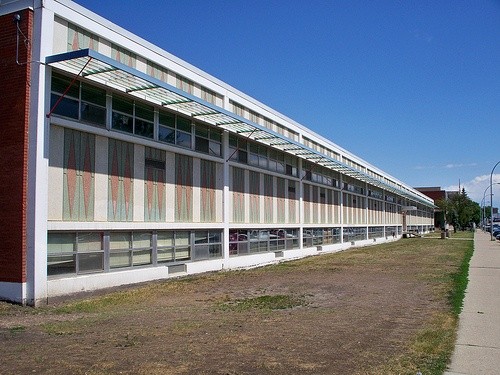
[483,224,491,230]
[487,222,500,240]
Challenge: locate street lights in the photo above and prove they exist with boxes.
[480,193,494,229]
[484,182,500,233]
